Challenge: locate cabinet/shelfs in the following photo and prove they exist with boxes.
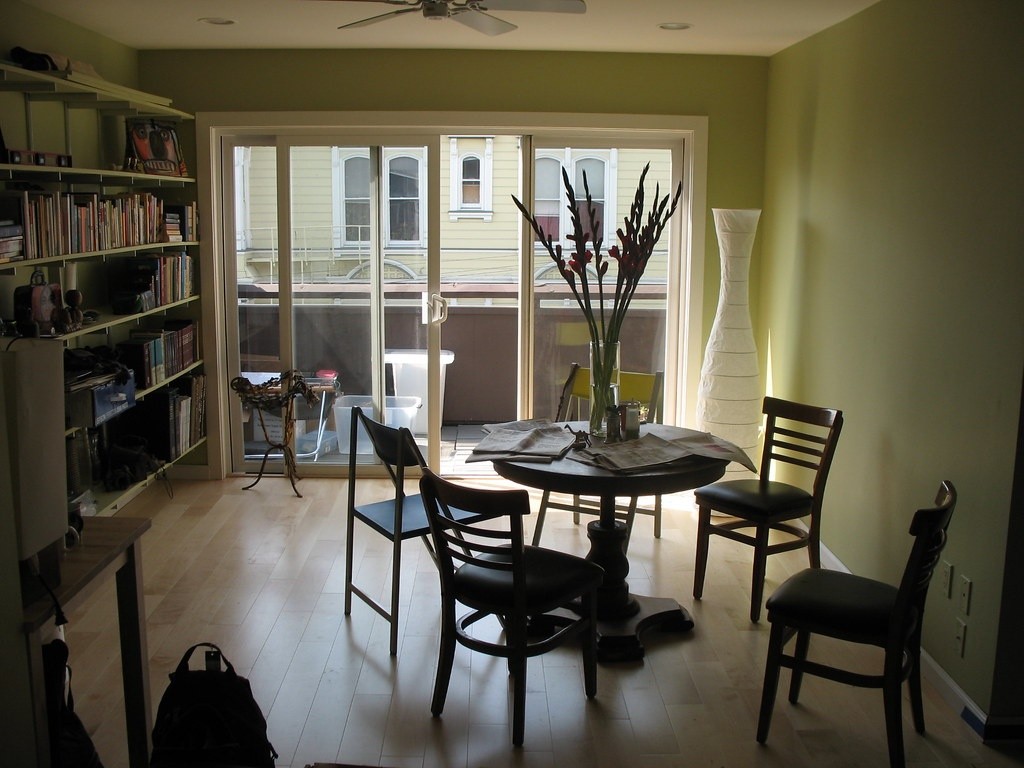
[0,61,208,517]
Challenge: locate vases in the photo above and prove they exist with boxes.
[588,340,621,443]
[698,207,763,517]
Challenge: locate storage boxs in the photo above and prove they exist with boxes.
[332,395,422,454]
[297,431,336,458]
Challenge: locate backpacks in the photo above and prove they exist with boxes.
[149,642,279,768]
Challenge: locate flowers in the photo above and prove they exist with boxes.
[510,162,684,428]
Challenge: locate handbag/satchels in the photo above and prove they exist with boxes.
[13,270,63,336]
[108,434,174,499]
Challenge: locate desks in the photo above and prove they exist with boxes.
[19,516,154,768]
[493,421,732,661]
[244,385,336,462]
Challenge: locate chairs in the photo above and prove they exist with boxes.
[757,479,959,768]
[530,363,664,556]
[419,466,606,747]
[694,395,844,625]
[343,404,505,658]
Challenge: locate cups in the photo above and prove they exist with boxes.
[621,400,640,431]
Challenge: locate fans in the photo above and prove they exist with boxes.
[337,0,586,37]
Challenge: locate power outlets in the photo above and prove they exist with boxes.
[941,560,953,598]
[954,617,967,657]
[959,574,972,615]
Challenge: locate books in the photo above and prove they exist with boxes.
[118,317,200,389]
[108,252,196,307]
[143,373,205,463]
[0,190,198,264]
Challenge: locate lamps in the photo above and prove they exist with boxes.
[421,3,448,21]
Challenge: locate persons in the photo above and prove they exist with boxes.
[32,284,62,322]
